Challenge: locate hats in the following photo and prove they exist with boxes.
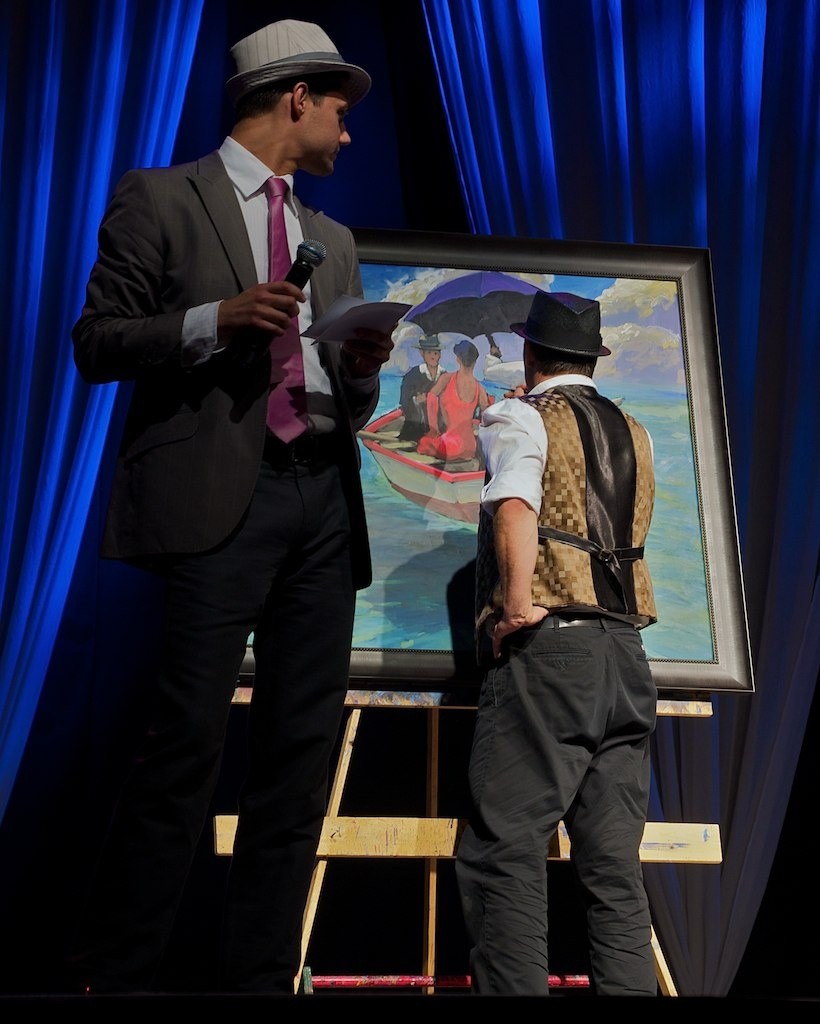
[225,19,372,109]
[510,289,611,356]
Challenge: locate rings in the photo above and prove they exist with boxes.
[355,356,361,365]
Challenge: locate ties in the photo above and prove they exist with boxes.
[266,176,309,444]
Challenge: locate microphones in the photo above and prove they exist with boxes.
[247,238,327,364]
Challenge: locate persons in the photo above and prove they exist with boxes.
[71,18,399,993]
[451,291,657,997]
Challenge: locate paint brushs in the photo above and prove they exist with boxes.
[494,386,529,394]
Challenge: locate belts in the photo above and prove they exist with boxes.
[519,614,634,628]
[268,433,328,465]
